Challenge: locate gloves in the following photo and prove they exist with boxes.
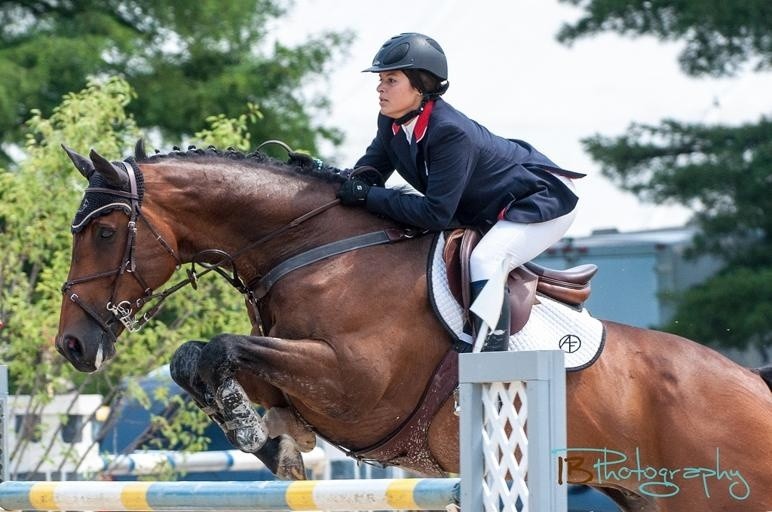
[334,176,370,207]
[284,150,318,171]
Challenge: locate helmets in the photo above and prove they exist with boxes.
[359,31,449,81]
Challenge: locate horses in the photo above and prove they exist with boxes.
[54,137,772,512]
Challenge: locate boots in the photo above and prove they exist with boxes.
[452,278,511,407]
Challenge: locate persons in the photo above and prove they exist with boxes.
[287,32,590,417]
